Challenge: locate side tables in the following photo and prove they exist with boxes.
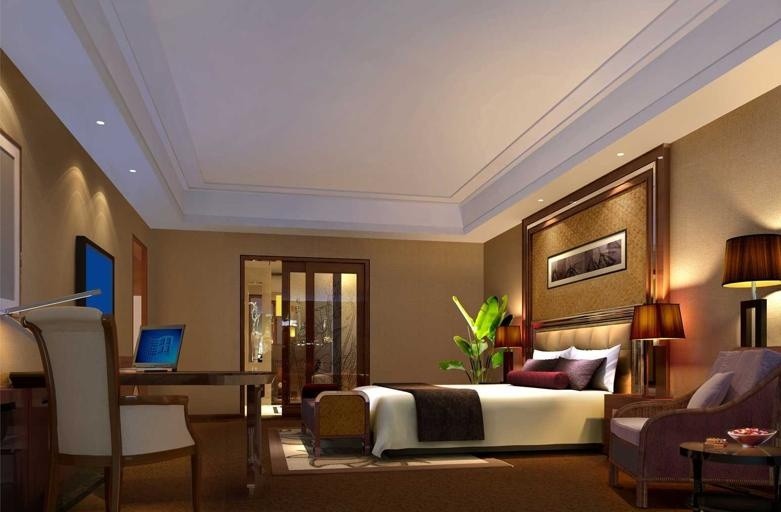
[679,441,780,512]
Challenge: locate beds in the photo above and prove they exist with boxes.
[352,320,632,459]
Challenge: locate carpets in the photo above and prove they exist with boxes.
[265,425,515,477]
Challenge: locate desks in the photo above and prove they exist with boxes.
[1,389,33,511]
[9,372,277,500]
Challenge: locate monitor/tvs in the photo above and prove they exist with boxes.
[75,236,115,320]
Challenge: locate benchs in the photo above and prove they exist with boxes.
[301,384,370,457]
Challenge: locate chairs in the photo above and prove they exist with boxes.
[20,307,204,511]
[608,349,781,512]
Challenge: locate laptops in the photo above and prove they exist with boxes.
[122,324,186,372]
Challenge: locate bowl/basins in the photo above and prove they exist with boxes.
[726,427,778,448]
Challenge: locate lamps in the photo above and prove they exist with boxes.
[721,233,780,347]
[494,326,523,383]
[630,303,686,399]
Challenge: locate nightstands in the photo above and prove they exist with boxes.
[604,394,674,457]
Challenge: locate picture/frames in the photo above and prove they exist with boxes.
[76,235,115,307]
[521,142,670,329]
[0,128,22,316]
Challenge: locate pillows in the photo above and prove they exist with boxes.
[687,348,778,410]
[506,345,622,393]
